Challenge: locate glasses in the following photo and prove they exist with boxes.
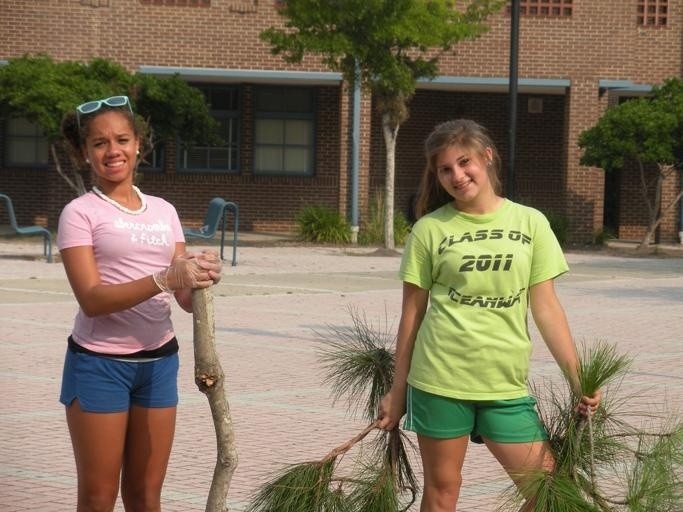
[72,96,137,133]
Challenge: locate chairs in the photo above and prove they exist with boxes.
[178,197,238,268]
[0,192,53,265]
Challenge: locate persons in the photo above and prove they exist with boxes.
[377,117,601,511]
[57,96,221,512]
